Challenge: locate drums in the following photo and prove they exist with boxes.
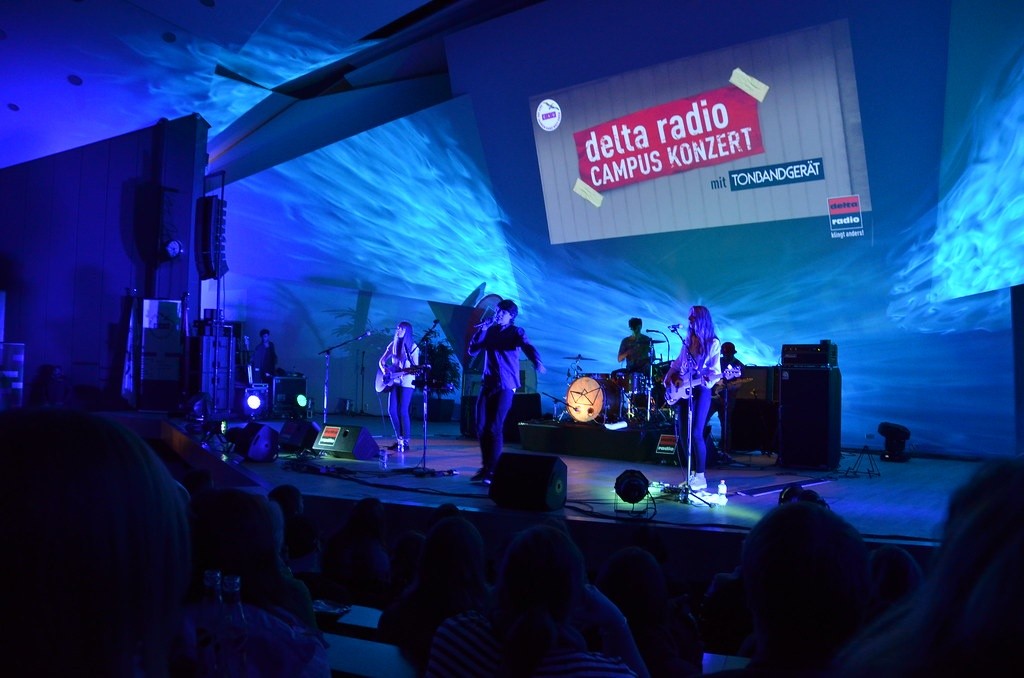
[565,360,676,425]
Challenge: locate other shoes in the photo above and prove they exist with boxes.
[387,441,398,450]
[394,445,409,451]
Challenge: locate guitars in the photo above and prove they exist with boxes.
[709,377,754,399]
[664,368,742,405]
[375,364,431,393]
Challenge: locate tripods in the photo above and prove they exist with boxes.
[649,328,716,508]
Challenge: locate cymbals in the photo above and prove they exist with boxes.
[561,356,598,361]
[631,339,666,344]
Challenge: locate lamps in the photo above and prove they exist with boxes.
[246,395,261,419]
[148,229,184,263]
[613,470,650,515]
[287,395,308,420]
[206,420,228,444]
[877,423,911,462]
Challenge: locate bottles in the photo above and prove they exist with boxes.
[397,436,404,452]
[718,480,727,506]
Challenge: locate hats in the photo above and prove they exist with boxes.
[720,342,737,354]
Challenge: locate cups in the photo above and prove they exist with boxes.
[378,447,387,463]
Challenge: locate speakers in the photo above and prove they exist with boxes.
[488,452,568,511]
[777,366,842,471]
[312,424,380,460]
[731,366,779,452]
[194,195,229,281]
[234,421,280,462]
[278,420,320,456]
[152,241,179,261]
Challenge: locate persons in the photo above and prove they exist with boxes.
[252,330,280,371]
[0,409,1024,678]
[664,306,721,489]
[705,342,744,451]
[618,318,656,379]
[378,321,419,452]
[468,300,547,481]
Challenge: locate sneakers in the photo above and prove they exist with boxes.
[468,468,490,483]
[484,472,493,483]
[679,475,694,487]
[690,477,707,490]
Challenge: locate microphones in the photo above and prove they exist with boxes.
[577,366,583,371]
[668,324,683,328]
[646,329,659,333]
[474,317,496,328]
[358,331,371,340]
[574,407,580,412]
